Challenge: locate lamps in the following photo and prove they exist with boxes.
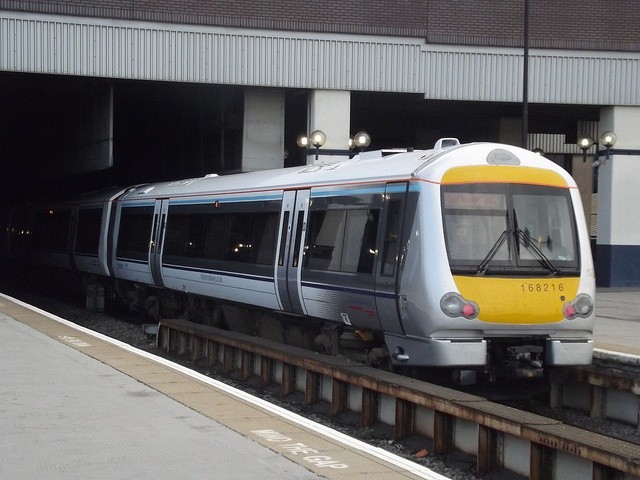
[577,135,594,150]
[349,135,356,150]
[309,130,327,149]
[296,132,312,150]
[533,147,545,156]
[600,130,618,147]
[354,131,372,148]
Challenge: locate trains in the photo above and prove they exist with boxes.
[0,137,596,414]
